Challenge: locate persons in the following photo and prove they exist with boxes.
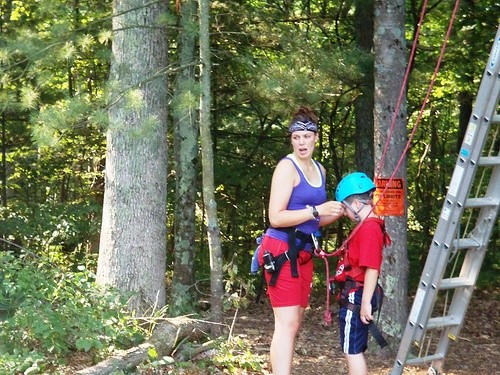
[256,107,345,375]
[332,172,387,375]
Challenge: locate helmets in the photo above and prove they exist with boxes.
[335,171,377,202]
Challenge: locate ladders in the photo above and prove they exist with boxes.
[390,22,500,375]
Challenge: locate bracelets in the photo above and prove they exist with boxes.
[312,205,319,220]
